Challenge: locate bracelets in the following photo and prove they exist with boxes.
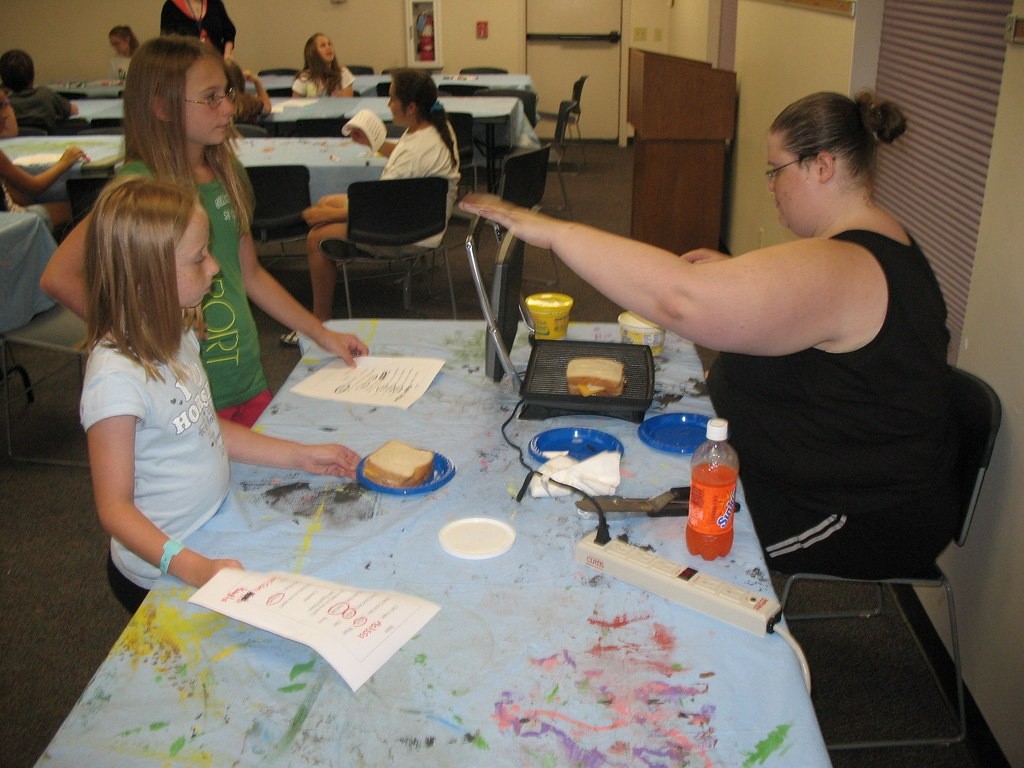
[159,539,185,574]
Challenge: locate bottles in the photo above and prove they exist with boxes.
[685,416,739,561]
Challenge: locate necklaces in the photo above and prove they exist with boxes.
[187,0,203,33]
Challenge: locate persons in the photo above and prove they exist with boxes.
[458,91,951,574]
[41,35,369,430]
[109,25,140,57]
[293,33,356,98]
[160,0,236,60]
[78,178,361,615]
[0,147,84,234]
[280,70,461,345]
[0,90,18,138]
[0,49,79,134]
[222,59,272,125]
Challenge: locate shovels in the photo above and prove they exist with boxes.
[575,487,741,517]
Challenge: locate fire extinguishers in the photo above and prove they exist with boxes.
[416,7,434,61]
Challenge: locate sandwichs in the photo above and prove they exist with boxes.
[566,357,624,396]
[364,440,435,488]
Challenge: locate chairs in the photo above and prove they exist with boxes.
[470,143,561,301]
[778,364,1004,748]
[498,101,583,218]
[534,75,583,150]
[326,177,460,321]
[241,164,316,293]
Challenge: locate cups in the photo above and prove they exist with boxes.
[618,312,666,356]
[524,292,574,340]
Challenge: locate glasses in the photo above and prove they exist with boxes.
[764,151,836,194]
[184,87,237,111]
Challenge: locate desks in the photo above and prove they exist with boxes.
[39,72,535,230]
[28,317,835,768]
[0,137,412,245]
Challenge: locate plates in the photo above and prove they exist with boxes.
[527,426,624,464]
[637,412,711,455]
[356,450,457,495]
